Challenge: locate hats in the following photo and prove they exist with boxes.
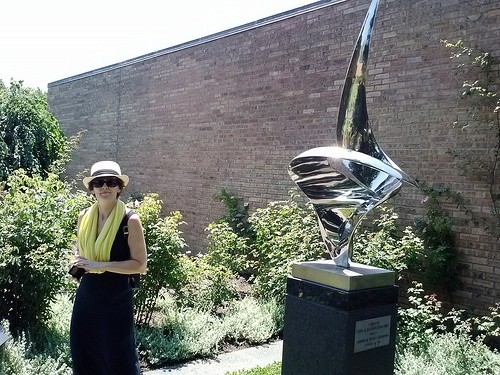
[83,161,129,189]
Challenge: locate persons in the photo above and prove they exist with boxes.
[68,160,148,375]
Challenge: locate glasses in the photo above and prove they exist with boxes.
[91,178,121,187]
